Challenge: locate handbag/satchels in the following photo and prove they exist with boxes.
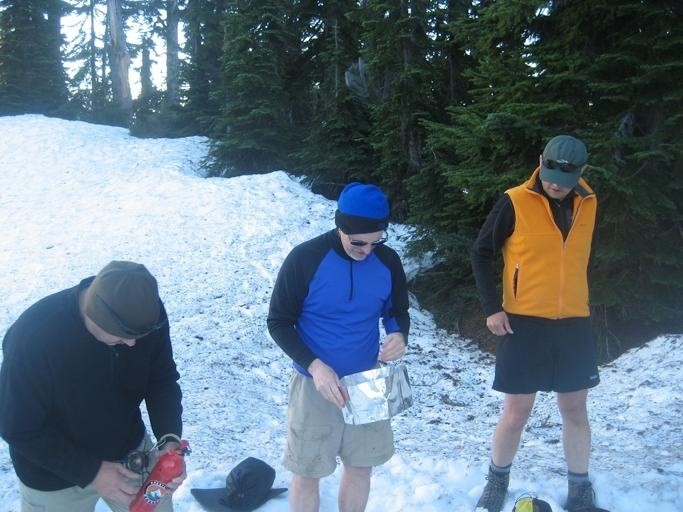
[219,456,277,510]
[514,497,552,512]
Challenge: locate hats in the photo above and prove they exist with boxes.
[334,182,390,234]
[83,261,159,340]
[538,135,588,188]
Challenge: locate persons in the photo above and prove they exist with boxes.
[470,134,599,512]
[1,260,187,512]
[267,182,410,511]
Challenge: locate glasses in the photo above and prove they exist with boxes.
[542,153,587,173]
[347,230,388,247]
[90,291,167,337]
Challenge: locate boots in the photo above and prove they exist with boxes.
[473,466,509,512]
[566,479,612,512]
